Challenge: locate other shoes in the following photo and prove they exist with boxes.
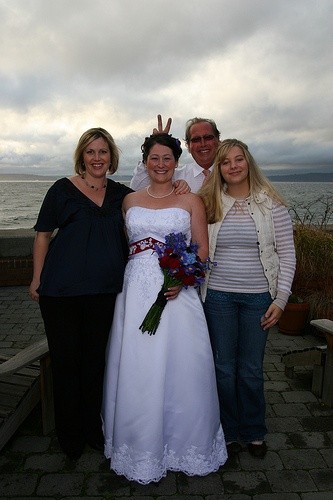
[82,430,104,450]
[63,438,81,461]
[251,440,267,458]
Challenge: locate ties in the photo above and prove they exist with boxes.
[202,170,211,178]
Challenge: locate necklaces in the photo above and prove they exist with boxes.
[81,172,107,191]
[146,183,176,198]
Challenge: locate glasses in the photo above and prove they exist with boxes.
[189,134,215,142]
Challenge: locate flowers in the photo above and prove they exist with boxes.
[139,231,218,336]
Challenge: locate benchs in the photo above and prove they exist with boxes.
[310,319,333,409]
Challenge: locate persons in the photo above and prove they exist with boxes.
[195,139,297,458]
[28,128,191,458]
[98,133,228,486]
[128,115,221,196]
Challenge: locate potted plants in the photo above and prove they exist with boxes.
[276,264,323,333]
